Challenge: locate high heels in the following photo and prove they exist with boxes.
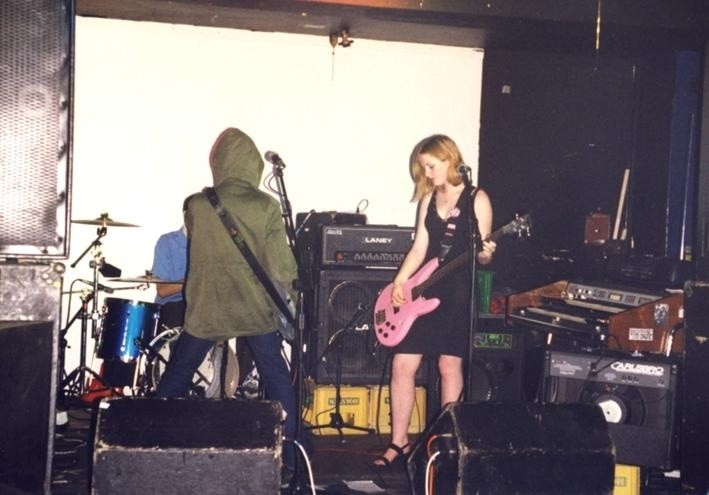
[372,442,411,470]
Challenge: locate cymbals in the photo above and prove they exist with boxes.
[108,279,185,285]
[69,218,140,227]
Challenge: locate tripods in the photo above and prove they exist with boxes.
[58,288,123,403]
[303,308,375,444]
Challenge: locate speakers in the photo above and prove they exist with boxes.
[0,319,56,495]
[425,328,524,425]
[90,395,283,494]
[403,402,616,495]
[304,265,429,385]
[540,347,679,471]
[679,281,709,495]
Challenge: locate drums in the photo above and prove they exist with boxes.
[142,327,239,402]
[95,297,164,366]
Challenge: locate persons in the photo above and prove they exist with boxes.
[153,128,314,481]
[147,190,203,366]
[369,134,495,470]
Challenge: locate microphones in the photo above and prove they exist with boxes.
[456,165,470,175]
[264,151,285,167]
[80,278,114,294]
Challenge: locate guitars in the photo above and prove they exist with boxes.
[373,213,534,348]
